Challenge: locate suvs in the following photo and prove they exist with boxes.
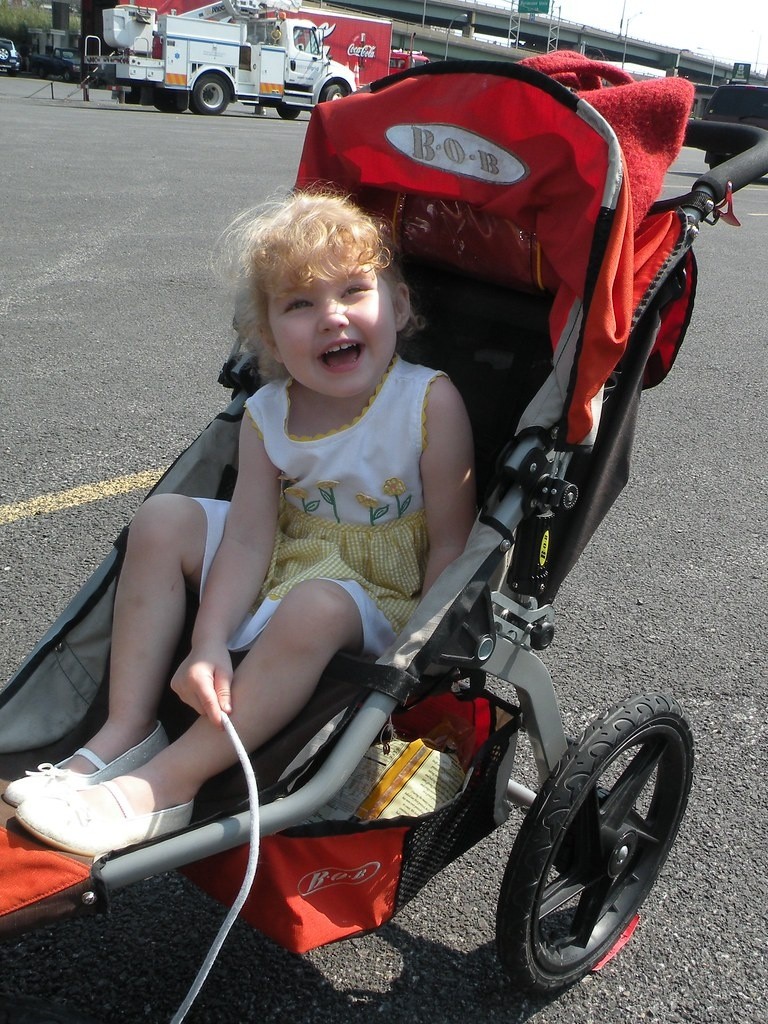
[701,80,767,174]
[0,38,21,76]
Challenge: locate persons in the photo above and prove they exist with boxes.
[3,194,475,856]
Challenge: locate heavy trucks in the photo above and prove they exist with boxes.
[118,0,431,91]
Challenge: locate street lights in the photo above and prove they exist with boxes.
[445,13,468,60]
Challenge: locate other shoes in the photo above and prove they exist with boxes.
[13,781,194,857]
[2,718,169,808]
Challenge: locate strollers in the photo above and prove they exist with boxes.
[0,49,768,998]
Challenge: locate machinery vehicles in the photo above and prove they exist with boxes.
[102,4,357,120]
